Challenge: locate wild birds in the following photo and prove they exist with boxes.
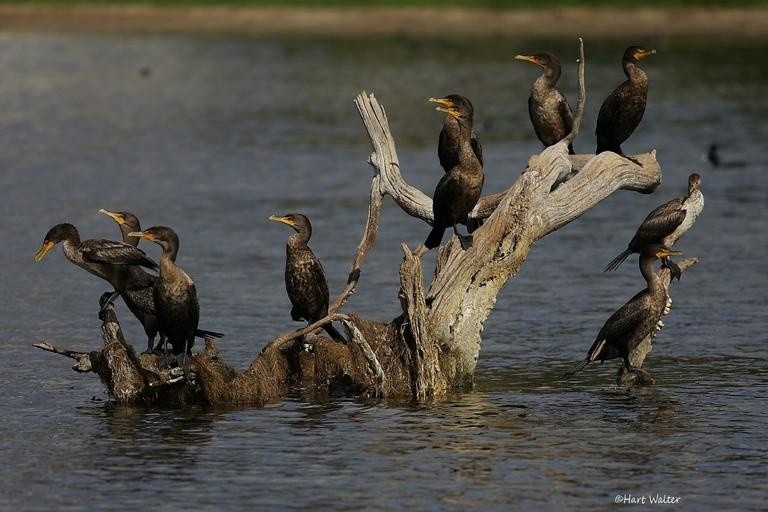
[514,52,576,155]
[268,212,348,346]
[424,104,486,252]
[603,173,706,283]
[595,45,657,168]
[556,243,685,380]
[34,207,225,378]
[428,94,484,174]
